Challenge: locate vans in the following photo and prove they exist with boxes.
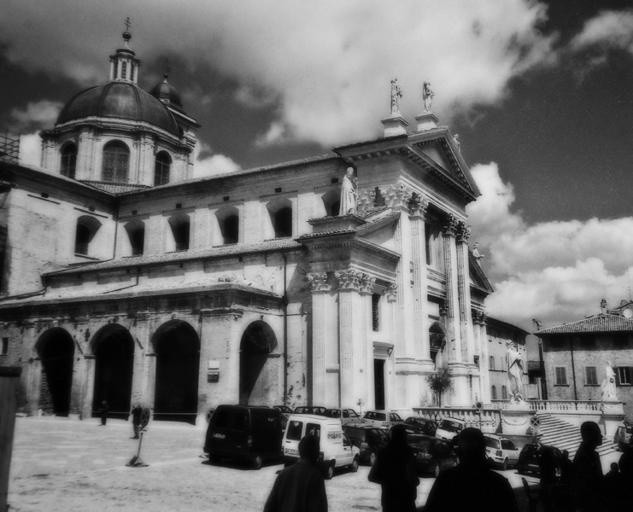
[204,405,282,469]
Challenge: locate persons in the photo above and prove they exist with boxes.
[99,400,107,426]
[130,402,141,439]
[568,420,605,511]
[606,461,619,476]
[423,426,517,511]
[366,423,419,511]
[259,434,327,511]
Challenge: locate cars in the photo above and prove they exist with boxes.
[281,406,467,479]
[483,433,521,470]
[517,444,573,481]
